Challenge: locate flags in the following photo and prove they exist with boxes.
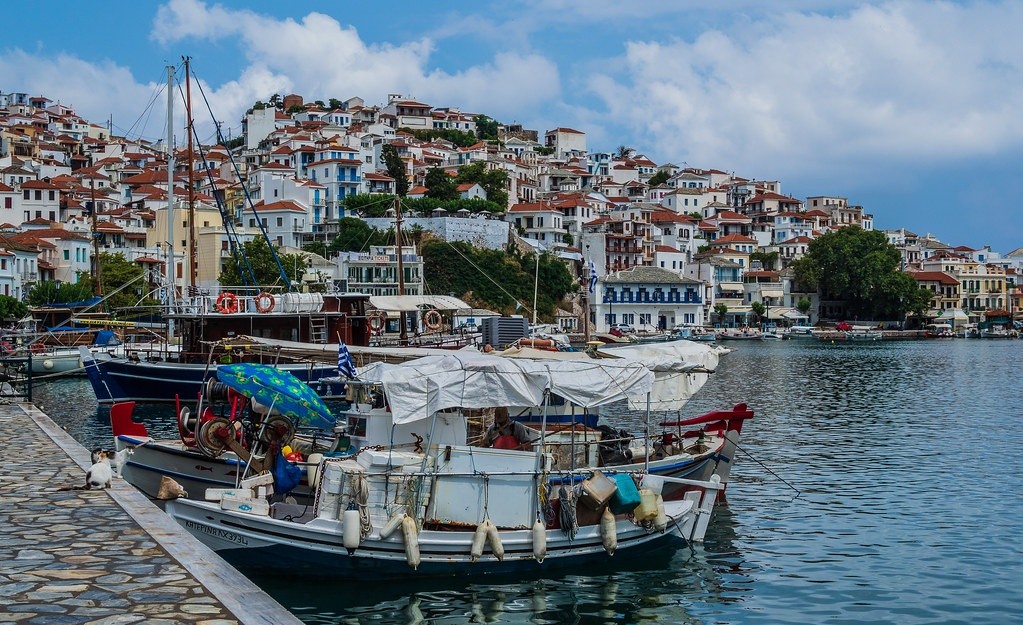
[588,257,597,293]
[337,340,356,379]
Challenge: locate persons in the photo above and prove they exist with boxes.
[943,328,946,333]
[795,319,798,326]
[879,322,883,330]
[477,407,531,451]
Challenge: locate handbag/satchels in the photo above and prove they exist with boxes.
[276,439,301,495]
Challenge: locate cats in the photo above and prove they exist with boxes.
[91,446,135,478]
[56,451,112,491]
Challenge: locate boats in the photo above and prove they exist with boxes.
[926,324,1023,338]
[595,326,883,341]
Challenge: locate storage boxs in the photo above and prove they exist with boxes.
[219,495,270,515]
[205,487,250,500]
[241,470,275,489]
[318,450,436,506]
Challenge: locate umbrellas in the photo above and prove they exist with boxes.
[217,362,336,489]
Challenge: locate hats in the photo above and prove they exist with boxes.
[494,404,508,423]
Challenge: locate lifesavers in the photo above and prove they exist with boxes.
[520,339,556,346]
[217,292,237,314]
[35,343,44,352]
[255,292,275,313]
[425,310,442,329]
[368,314,384,331]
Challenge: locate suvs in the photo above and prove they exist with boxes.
[612,323,635,333]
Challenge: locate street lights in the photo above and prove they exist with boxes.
[765,296,770,326]
[136,286,142,307]
[608,289,613,326]
[55,279,62,304]
[0,54,756,580]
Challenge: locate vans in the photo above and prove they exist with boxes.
[673,323,695,334]
